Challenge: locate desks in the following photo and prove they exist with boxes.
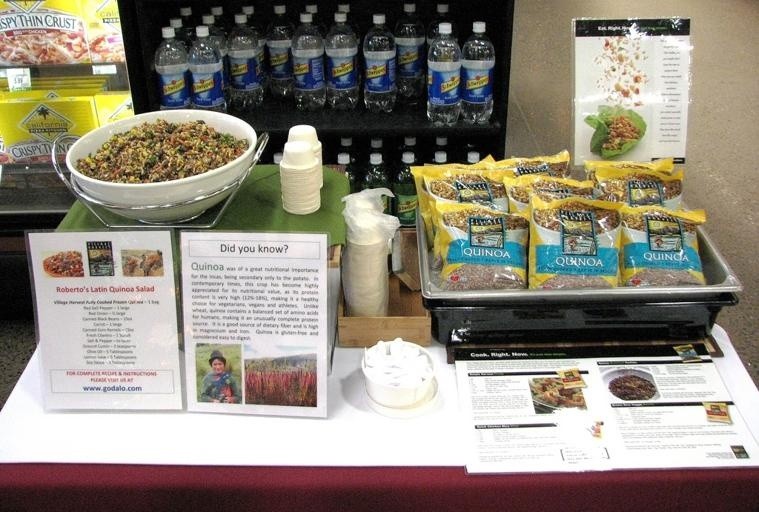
[0,349,759,512]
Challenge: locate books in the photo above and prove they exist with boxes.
[454,355,759,475]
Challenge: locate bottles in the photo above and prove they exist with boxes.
[335,134,485,227]
[153,4,453,112]
[425,23,496,126]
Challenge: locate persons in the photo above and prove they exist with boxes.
[200,349,240,403]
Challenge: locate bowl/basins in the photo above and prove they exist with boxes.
[64,110,258,223]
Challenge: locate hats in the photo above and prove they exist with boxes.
[209,350,227,366]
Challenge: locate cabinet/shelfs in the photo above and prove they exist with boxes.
[116,0,517,228]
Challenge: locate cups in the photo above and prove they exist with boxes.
[347,216,386,315]
[275,124,325,217]
